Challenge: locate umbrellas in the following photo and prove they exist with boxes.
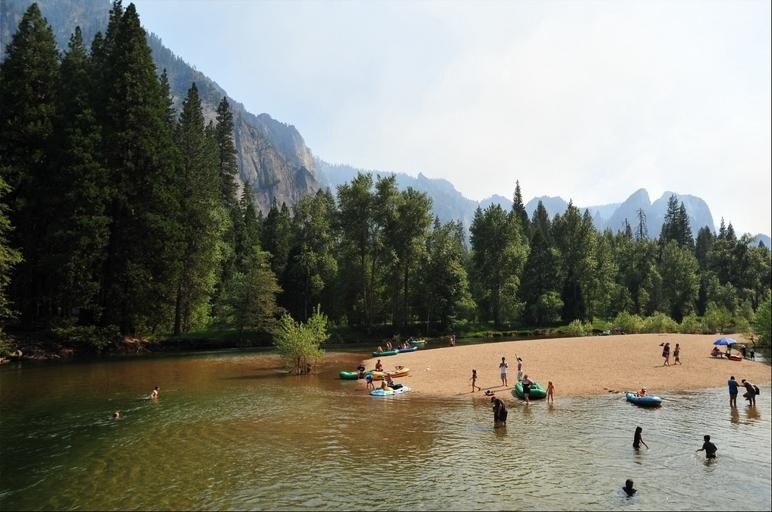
[713,337,737,345]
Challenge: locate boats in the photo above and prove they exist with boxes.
[339,369,366,380]
[728,354,743,361]
[625,391,662,407]
[368,365,410,380]
[408,340,426,344]
[369,383,410,395]
[398,346,418,352]
[372,348,399,356]
[515,379,547,400]
[413,343,425,348]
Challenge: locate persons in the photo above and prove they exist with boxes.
[357,359,403,390]
[622,478,637,497]
[662,343,670,366]
[728,376,760,408]
[637,387,648,397]
[673,343,681,365]
[113,407,120,418]
[710,343,755,361]
[151,386,160,399]
[633,426,649,451]
[468,353,555,428]
[696,435,718,459]
[376,334,455,353]
